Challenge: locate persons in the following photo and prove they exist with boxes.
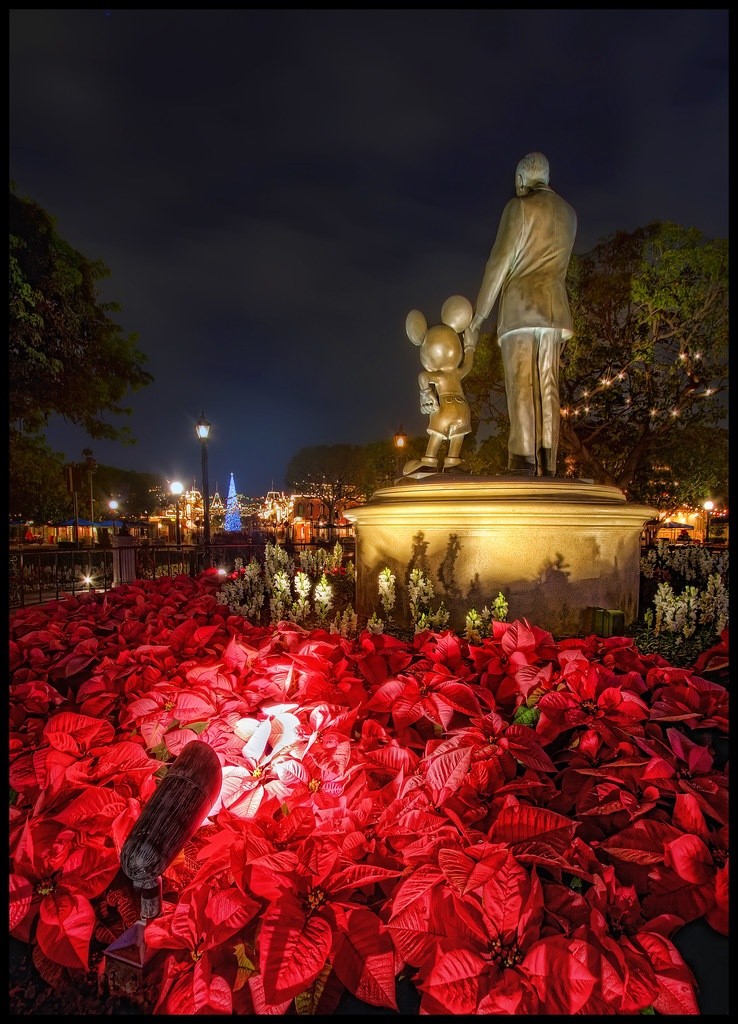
[470,152,577,478]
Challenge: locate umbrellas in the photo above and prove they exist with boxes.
[9,519,154,544]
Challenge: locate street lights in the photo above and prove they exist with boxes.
[109,500,119,536]
[194,410,212,571]
[171,482,183,563]
[704,501,713,541]
[395,424,407,482]
